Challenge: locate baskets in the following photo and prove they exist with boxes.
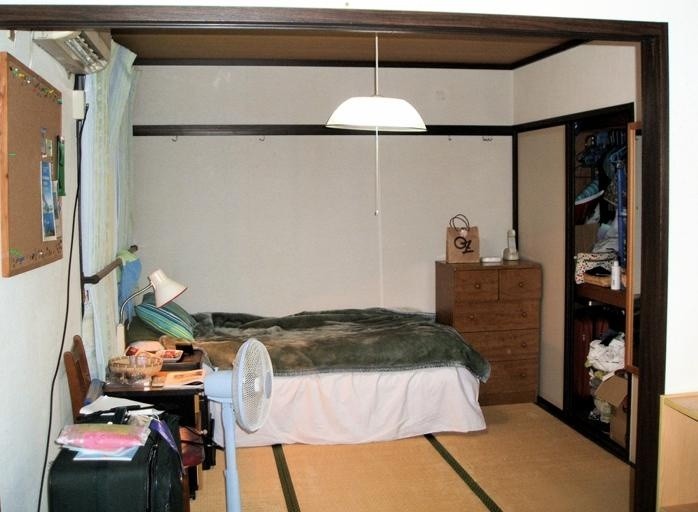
[108,355,164,377]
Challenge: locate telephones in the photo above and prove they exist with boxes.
[503,229,520,261]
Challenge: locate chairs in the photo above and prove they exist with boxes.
[63,335,192,512]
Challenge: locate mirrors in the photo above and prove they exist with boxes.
[625,122,643,377]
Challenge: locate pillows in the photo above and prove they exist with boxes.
[134,292,199,343]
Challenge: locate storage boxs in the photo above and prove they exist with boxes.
[593,373,628,450]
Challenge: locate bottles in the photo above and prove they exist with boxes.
[84,378,103,405]
[611,260,621,291]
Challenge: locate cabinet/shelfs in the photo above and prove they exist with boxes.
[435,258,543,406]
[563,101,635,466]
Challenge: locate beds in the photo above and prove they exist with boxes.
[125,308,492,449]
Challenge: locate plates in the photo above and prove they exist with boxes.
[155,349,184,363]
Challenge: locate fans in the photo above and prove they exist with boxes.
[204,338,274,512]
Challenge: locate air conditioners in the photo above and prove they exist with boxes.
[32,26,111,74]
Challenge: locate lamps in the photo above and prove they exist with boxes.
[325,33,428,133]
[117,267,189,354]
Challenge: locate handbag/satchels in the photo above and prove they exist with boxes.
[446,214,480,263]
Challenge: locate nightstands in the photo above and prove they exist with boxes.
[104,368,204,490]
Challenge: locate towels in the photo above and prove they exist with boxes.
[117,251,142,331]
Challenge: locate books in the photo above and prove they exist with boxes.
[162,368,207,390]
[61,443,128,454]
[67,446,137,457]
[71,446,141,462]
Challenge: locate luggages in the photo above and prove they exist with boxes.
[48,404,183,512]
